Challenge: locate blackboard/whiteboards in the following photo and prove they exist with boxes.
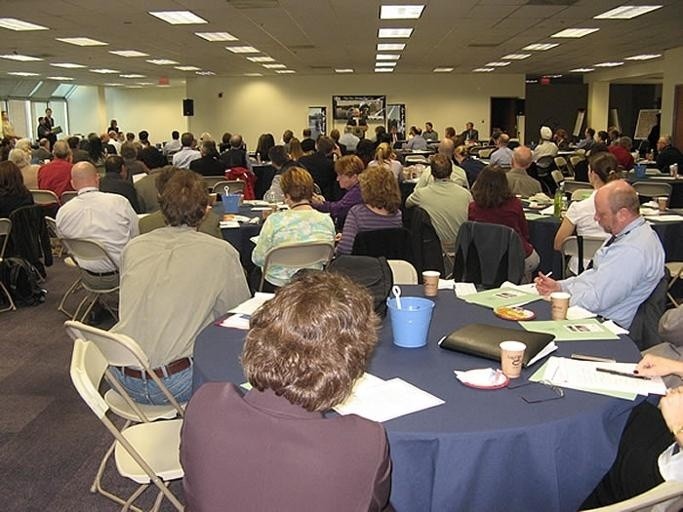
[633,109,661,140]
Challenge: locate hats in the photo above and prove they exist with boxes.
[540,126,552,140]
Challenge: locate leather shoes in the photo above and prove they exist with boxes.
[88,309,113,325]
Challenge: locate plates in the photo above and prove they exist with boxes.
[465,368,510,389]
[494,307,536,320]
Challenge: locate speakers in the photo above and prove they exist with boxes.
[182,99,193,116]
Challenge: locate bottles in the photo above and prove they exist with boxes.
[554,187,560,217]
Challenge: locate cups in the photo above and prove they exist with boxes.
[549,291,570,320]
[422,271,440,296]
[221,194,240,213]
[385,296,436,349]
[496,339,526,380]
[658,197,668,212]
[632,164,646,177]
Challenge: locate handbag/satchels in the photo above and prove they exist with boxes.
[330,254,393,321]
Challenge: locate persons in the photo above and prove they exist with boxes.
[250,122,681,282]
[534,180,665,327]
[99,166,253,407]
[591,355,683,497]
[648,113,662,142]
[347,108,366,126]
[175,267,398,511]
[2,107,249,324]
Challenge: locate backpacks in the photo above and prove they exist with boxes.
[0,256,48,307]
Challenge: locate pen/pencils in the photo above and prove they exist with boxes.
[312,192,325,204]
[531,271,553,289]
[596,368,651,380]
[666,292,678,307]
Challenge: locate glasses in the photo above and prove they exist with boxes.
[508,380,564,404]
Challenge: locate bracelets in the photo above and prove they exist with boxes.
[672,426,682,436]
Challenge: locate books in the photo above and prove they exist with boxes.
[330,370,447,423]
[517,318,620,342]
[528,359,637,402]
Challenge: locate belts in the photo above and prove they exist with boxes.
[115,357,194,379]
[87,270,119,276]
